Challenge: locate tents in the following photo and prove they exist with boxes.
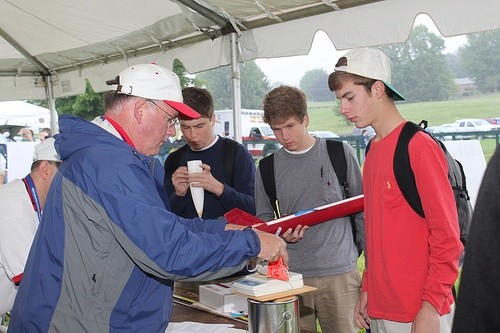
[0,101,58,135]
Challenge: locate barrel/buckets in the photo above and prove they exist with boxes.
[247,298,300,333]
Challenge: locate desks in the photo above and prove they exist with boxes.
[169,288,317,333]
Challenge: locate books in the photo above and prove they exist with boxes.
[199,269,304,313]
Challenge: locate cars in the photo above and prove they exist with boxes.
[453,119,500,140]
[425,126,439,140]
[439,124,454,140]
[309,131,340,139]
[352,126,376,138]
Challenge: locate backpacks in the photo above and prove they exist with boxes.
[365,119,474,267]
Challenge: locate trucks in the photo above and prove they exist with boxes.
[213,110,283,158]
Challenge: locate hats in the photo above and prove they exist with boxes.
[333,47,406,101]
[32,137,63,162]
[110,64,201,120]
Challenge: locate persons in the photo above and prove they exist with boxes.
[6,63,289,333]
[0,137,62,317]
[164,87,256,219]
[327,47,474,333]
[0,127,52,183]
[451,143,500,333]
[254,85,365,333]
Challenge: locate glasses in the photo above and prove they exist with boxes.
[145,99,177,128]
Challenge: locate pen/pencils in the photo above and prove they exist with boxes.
[274,226,282,236]
